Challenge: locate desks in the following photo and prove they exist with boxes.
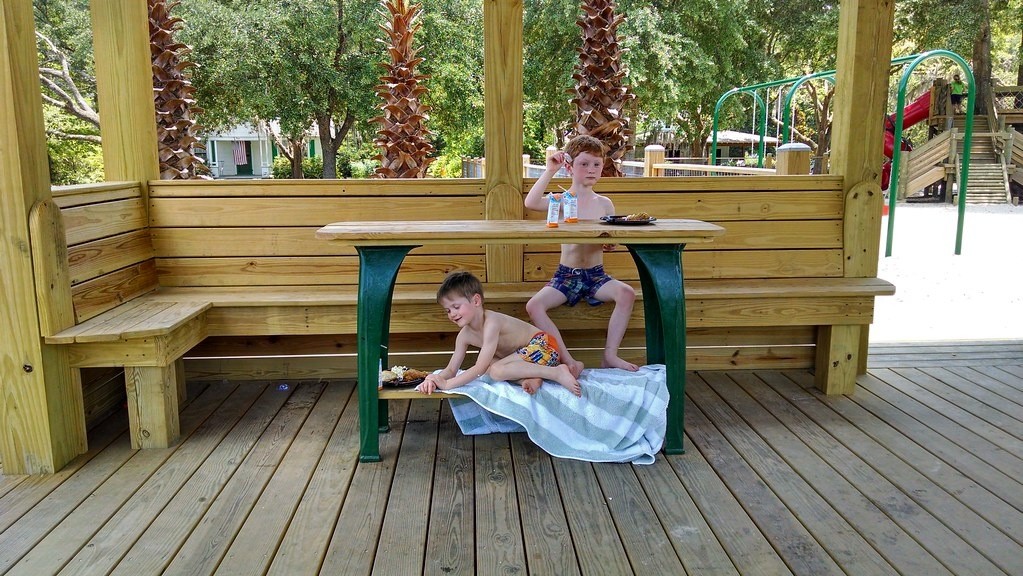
[315,218,726,463]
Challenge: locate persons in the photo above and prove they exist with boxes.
[526,134,639,379]
[950,75,966,115]
[414,271,582,399]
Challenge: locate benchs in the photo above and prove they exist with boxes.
[50,175,896,449]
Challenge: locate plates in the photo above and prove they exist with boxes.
[599,215,658,225]
[381,367,425,387]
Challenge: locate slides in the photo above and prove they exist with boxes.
[880,88,931,192]
